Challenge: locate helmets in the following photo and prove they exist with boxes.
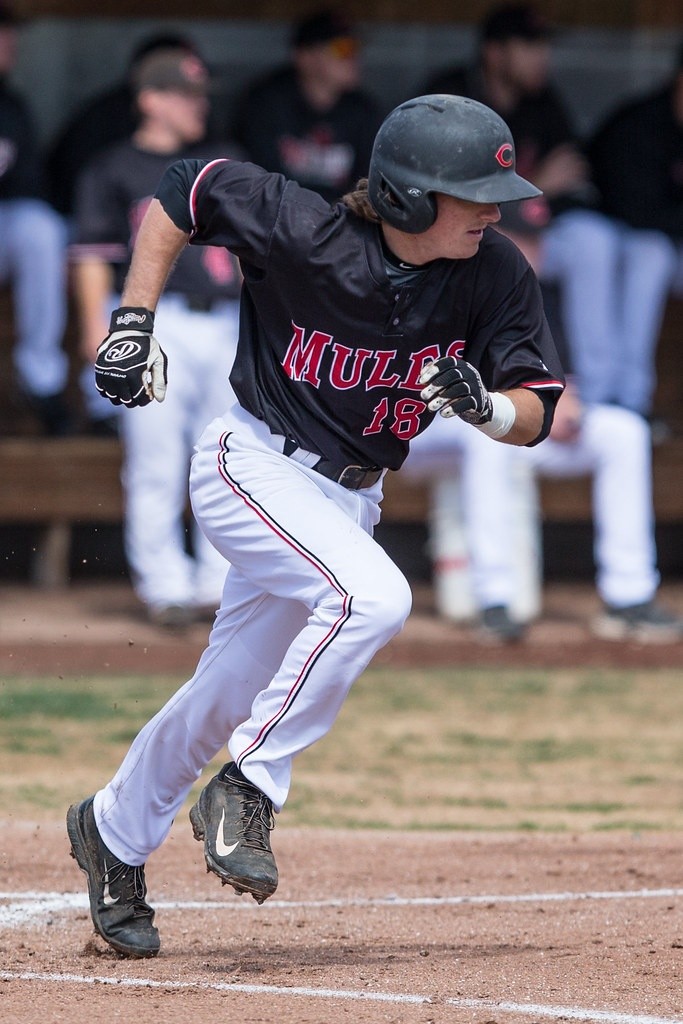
[367,94,544,234]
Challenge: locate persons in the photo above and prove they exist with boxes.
[67,46,243,625]
[571,70,683,297]
[67,94,567,960]
[0,0,67,431]
[421,12,673,423]
[224,13,387,206]
[409,199,682,647]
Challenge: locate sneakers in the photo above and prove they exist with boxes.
[66,795,159,958]
[189,761,279,904]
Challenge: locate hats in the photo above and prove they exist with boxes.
[136,48,208,97]
[475,10,563,42]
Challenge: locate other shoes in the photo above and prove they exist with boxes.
[189,603,222,621]
[148,607,186,631]
[596,605,680,648]
[454,609,526,645]
[27,393,73,435]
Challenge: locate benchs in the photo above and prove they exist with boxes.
[0,280,683,588]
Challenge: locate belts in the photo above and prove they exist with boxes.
[283,440,383,491]
[187,291,209,312]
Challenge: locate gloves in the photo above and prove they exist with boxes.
[94,306,167,409]
[417,356,492,426]
